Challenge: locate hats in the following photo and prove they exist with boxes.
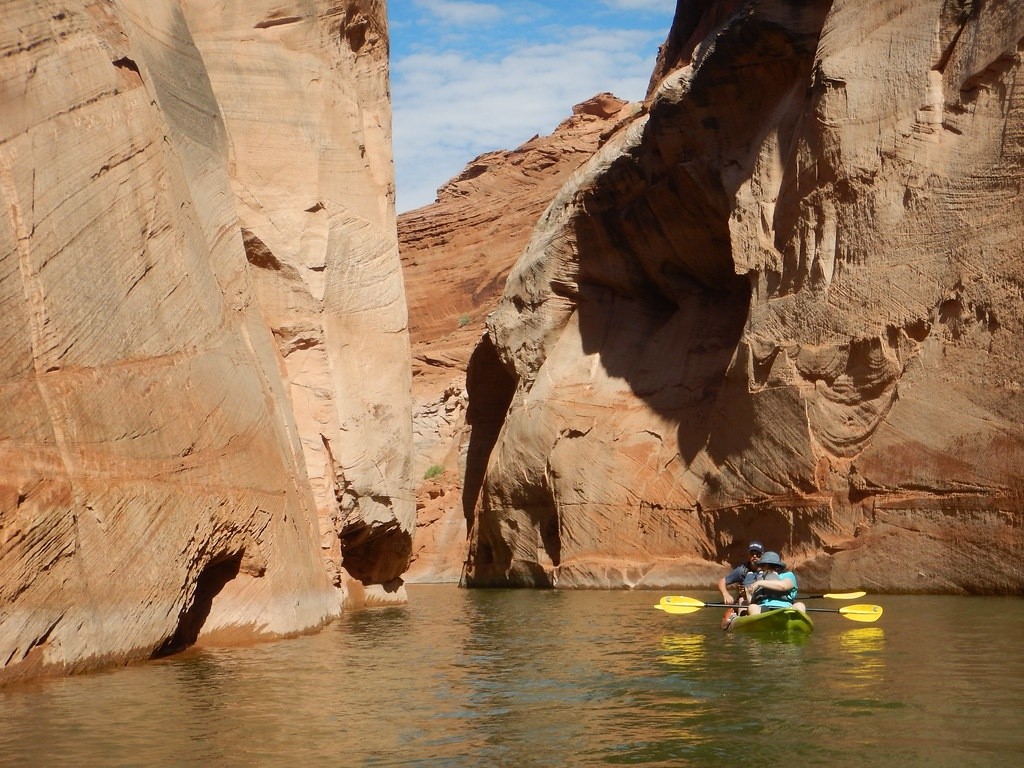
[748,541,765,553]
[757,550,786,569]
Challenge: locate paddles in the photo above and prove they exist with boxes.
[653,591,884,623]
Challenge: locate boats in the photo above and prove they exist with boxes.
[720,605,816,634]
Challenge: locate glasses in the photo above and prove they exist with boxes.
[751,551,761,557]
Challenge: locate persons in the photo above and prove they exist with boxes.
[717,541,764,616]
[747,552,806,615]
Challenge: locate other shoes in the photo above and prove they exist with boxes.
[761,606,771,613]
[740,611,749,617]
